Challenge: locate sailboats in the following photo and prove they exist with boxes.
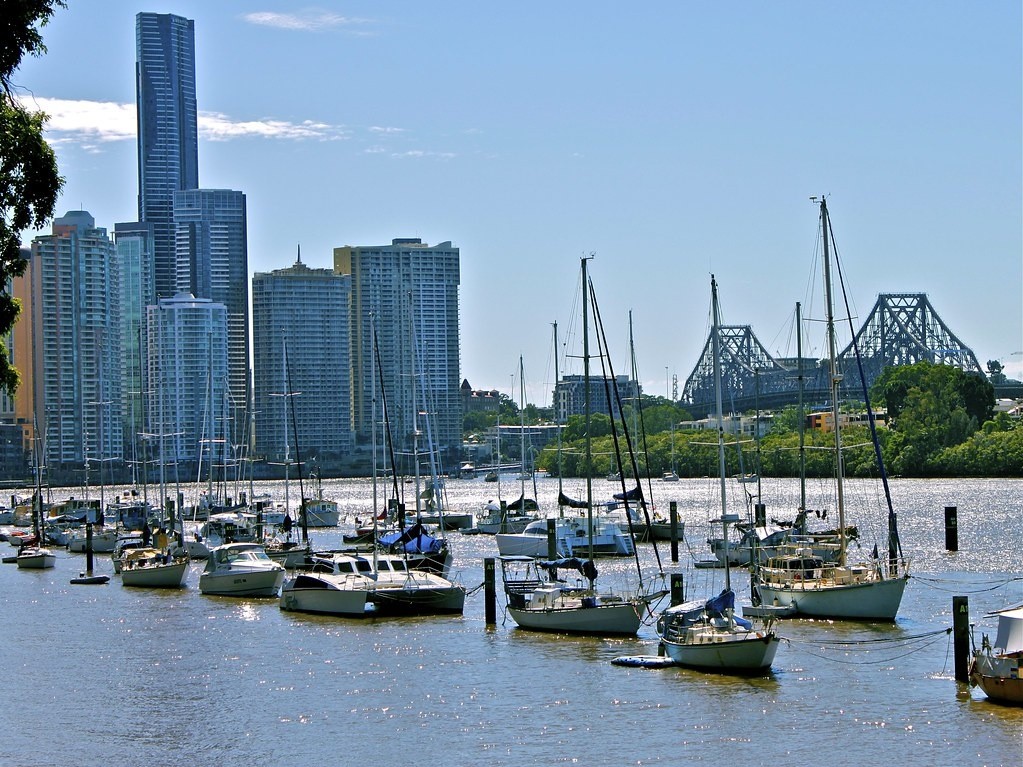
[476,353,543,535]
[495,250,670,638]
[278,311,466,618]
[611,311,684,540]
[16,411,56,570]
[654,272,782,674]
[747,194,913,624]
[496,320,635,560]
[707,368,860,569]
[0,240,296,597]
[344,288,474,540]
[664,416,680,481]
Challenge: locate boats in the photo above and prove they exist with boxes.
[517,473,532,480]
[736,472,758,482]
[295,466,340,528]
[461,464,475,480]
[608,473,623,480]
[484,471,498,483]
[969,598,1023,703]
